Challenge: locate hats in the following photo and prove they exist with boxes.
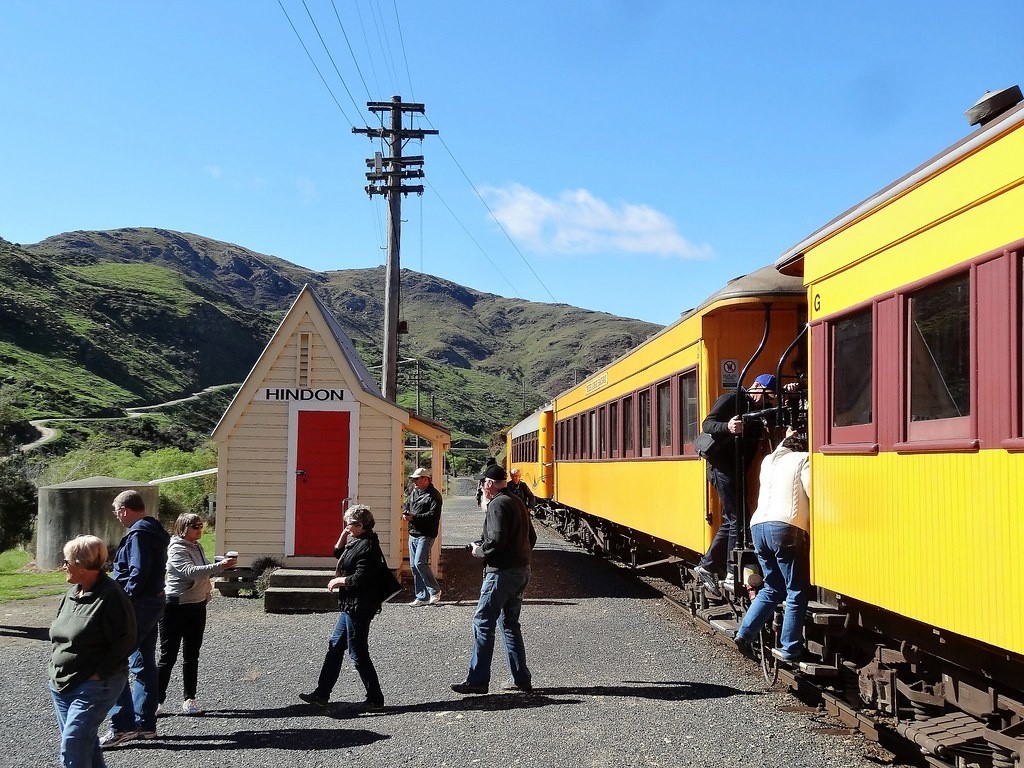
[474,465,508,480]
[756,374,777,397]
[408,468,431,477]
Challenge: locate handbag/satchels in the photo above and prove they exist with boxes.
[694,430,721,461]
[358,544,400,604]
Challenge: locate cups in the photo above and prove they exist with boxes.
[226,551,238,565]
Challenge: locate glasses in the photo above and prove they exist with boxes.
[113,510,124,515]
[185,523,205,529]
[64,559,81,567]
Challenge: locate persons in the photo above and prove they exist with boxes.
[296,504,384,712]
[506,466,536,513]
[403,467,443,607]
[449,464,538,695]
[152,513,239,714]
[693,373,800,596]
[99,490,170,748]
[735,426,823,664]
[48,534,138,768]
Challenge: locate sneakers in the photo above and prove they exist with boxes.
[183,698,202,713]
[299,692,328,706]
[99,730,140,747]
[409,599,429,607]
[450,678,489,694]
[155,703,167,716]
[505,682,532,691]
[694,565,722,596]
[139,729,158,738]
[352,699,385,715]
[725,573,734,583]
[429,590,442,603]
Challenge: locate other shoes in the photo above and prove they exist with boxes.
[782,651,823,662]
[734,636,755,660]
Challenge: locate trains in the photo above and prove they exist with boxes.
[505,84,1024,764]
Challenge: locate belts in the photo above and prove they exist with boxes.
[157,590,166,596]
